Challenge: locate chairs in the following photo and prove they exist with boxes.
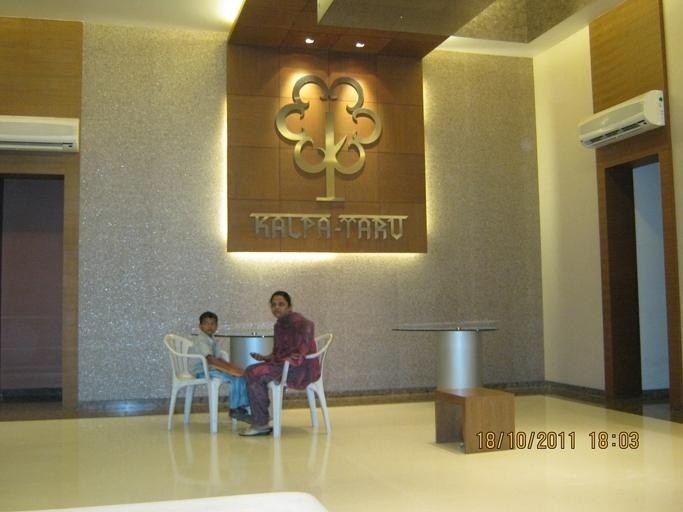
[262,333,333,447]
[163,334,237,432]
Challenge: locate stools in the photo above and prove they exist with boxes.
[433,386,519,453]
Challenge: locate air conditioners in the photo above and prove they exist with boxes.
[576,89,665,150]
[0,116,81,152]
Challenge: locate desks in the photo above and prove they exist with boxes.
[391,320,498,449]
[212,334,274,445]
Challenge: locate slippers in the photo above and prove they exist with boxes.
[239,426,273,436]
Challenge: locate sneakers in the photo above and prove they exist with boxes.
[229,407,252,424]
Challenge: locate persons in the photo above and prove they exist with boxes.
[187,311,252,426]
[239,290,321,437]
